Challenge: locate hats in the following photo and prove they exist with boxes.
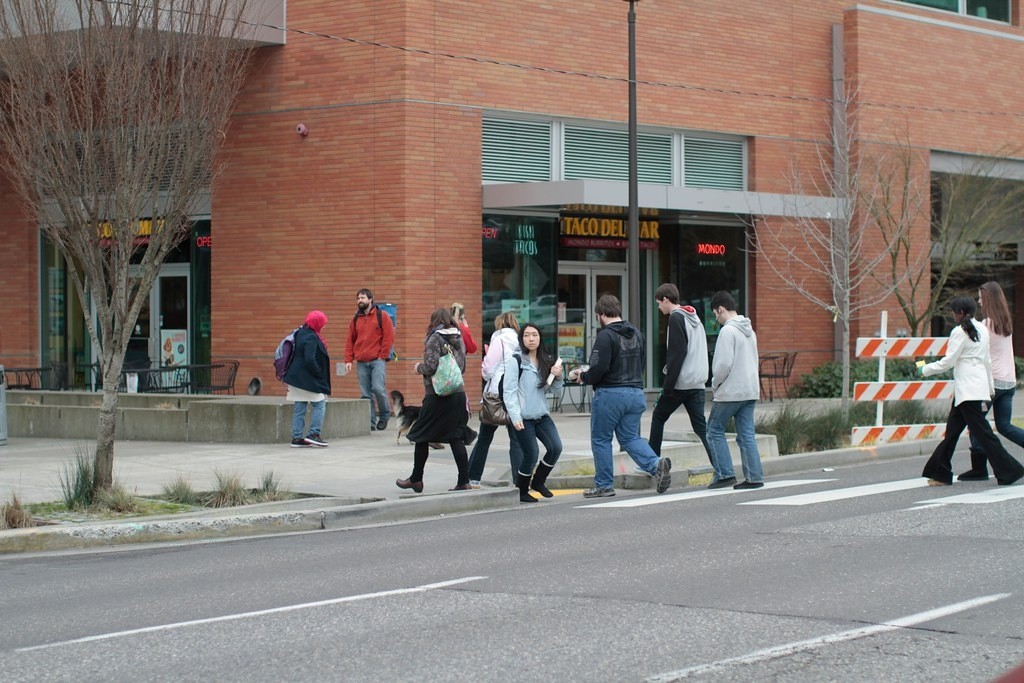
[305,311,328,332]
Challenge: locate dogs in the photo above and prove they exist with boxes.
[390,390,477,449]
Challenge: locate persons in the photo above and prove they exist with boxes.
[284,309,332,448]
[468,312,564,504]
[344,288,396,431]
[633,282,765,490]
[574,294,672,499]
[395,300,477,492]
[914,281,1024,485]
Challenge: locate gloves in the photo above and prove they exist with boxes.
[918,365,924,376]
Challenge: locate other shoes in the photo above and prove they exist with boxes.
[927,479,949,486]
[469,480,481,490]
[634,467,652,477]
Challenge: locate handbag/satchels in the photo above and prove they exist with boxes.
[479,392,511,426]
[428,335,465,397]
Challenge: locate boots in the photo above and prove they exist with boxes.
[530,459,554,498]
[515,470,538,502]
[957,447,989,481]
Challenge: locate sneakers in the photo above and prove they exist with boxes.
[583,488,615,498]
[304,436,328,446]
[656,457,672,493]
[708,477,736,489]
[733,481,763,489]
[290,439,313,447]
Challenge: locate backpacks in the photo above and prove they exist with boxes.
[498,354,524,413]
[354,303,397,361]
[274,326,303,382]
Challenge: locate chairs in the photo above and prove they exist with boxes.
[555,362,594,414]
[36,361,239,398]
[762,351,796,402]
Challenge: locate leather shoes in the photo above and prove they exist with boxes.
[371,427,375,431]
[396,478,423,493]
[448,485,472,491]
[377,420,387,430]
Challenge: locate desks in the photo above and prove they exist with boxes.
[164,363,226,395]
[122,368,163,392]
[72,364,103,392]
[4,367,38,391]
